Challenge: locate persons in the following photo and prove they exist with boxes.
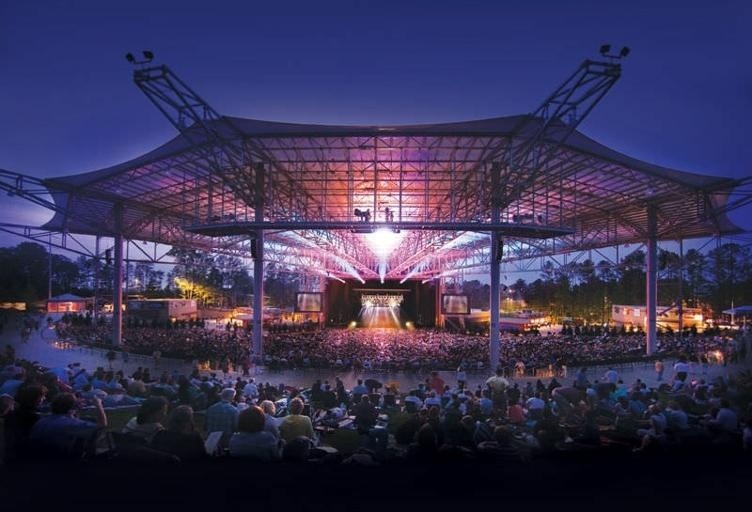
[0,311,752,511]
